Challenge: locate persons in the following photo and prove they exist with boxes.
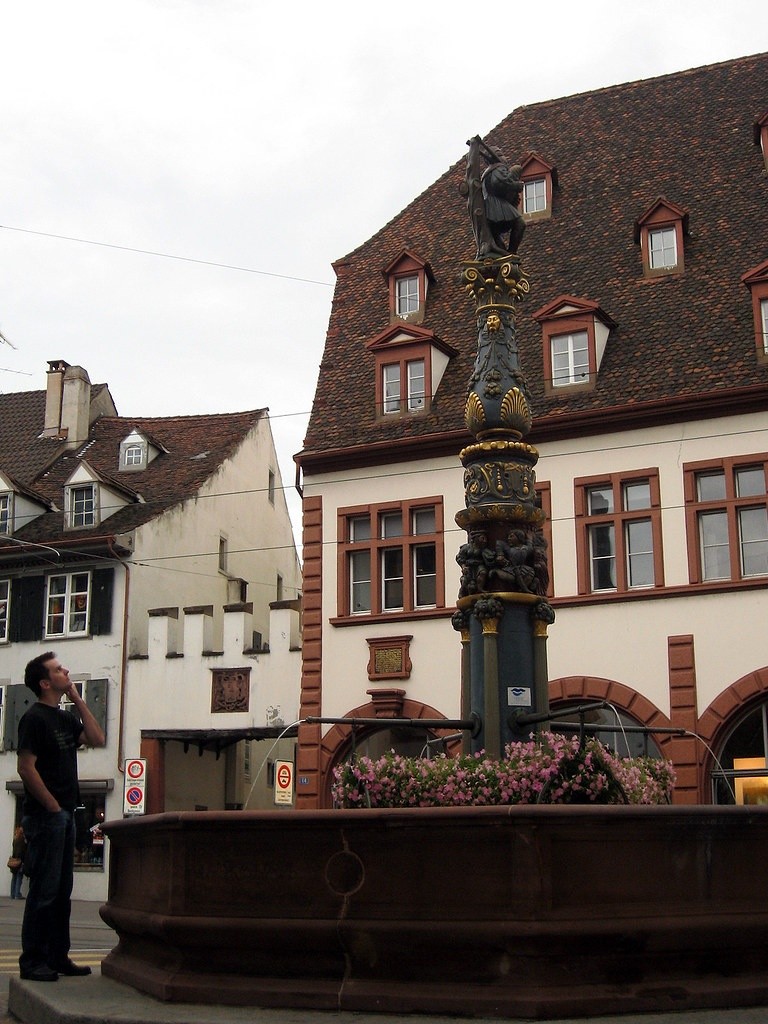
[16,651,105,981]
[486,310,501,331]
[481,146,527,255]
[456,529,550,596]
[10,827,26,899]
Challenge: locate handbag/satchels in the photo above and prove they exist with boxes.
[7,856,22,868]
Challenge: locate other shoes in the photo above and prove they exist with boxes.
[20,965,59,980]
[15,896,25,899]
[49,958,91,976]
[11,896,15,899]
